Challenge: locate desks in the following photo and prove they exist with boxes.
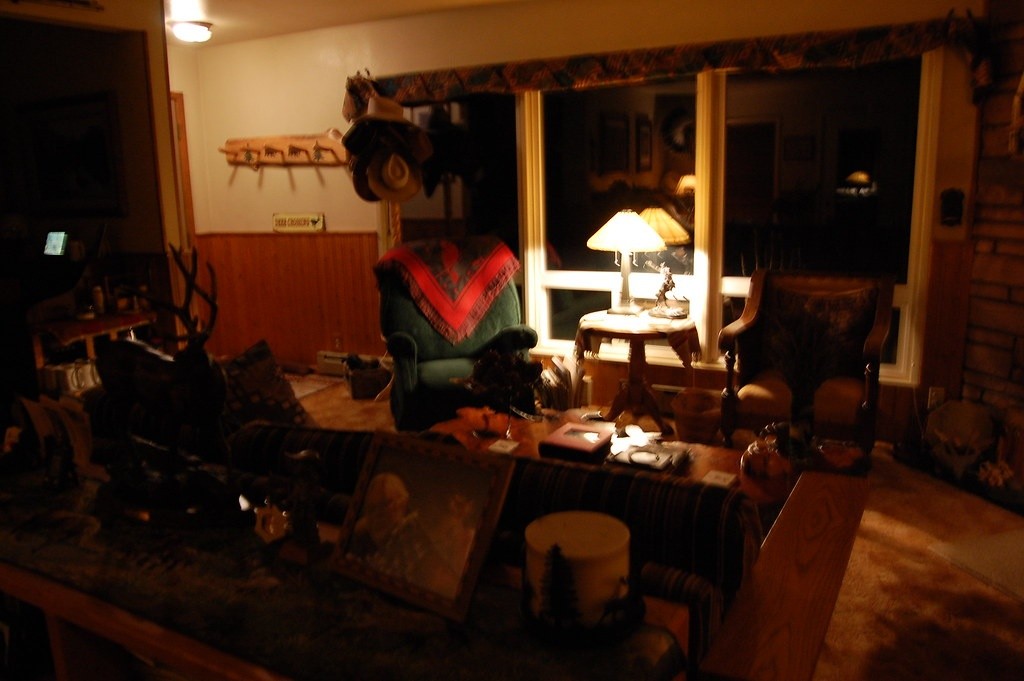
[574,305,704,438]
[50,302,158,365]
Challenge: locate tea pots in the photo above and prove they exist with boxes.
[739,435,790,498]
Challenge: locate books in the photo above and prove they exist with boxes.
[538,422,617,462]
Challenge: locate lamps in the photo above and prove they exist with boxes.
[634,206,690,273]
[587,207,663,314]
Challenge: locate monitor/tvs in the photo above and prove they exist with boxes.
[42,231,68,256]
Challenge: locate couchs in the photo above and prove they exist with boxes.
[371,234,543,433]
[717,268,891,467]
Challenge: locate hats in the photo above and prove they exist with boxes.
[341,97,433,204]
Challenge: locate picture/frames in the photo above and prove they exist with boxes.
[323,427,517,624]
